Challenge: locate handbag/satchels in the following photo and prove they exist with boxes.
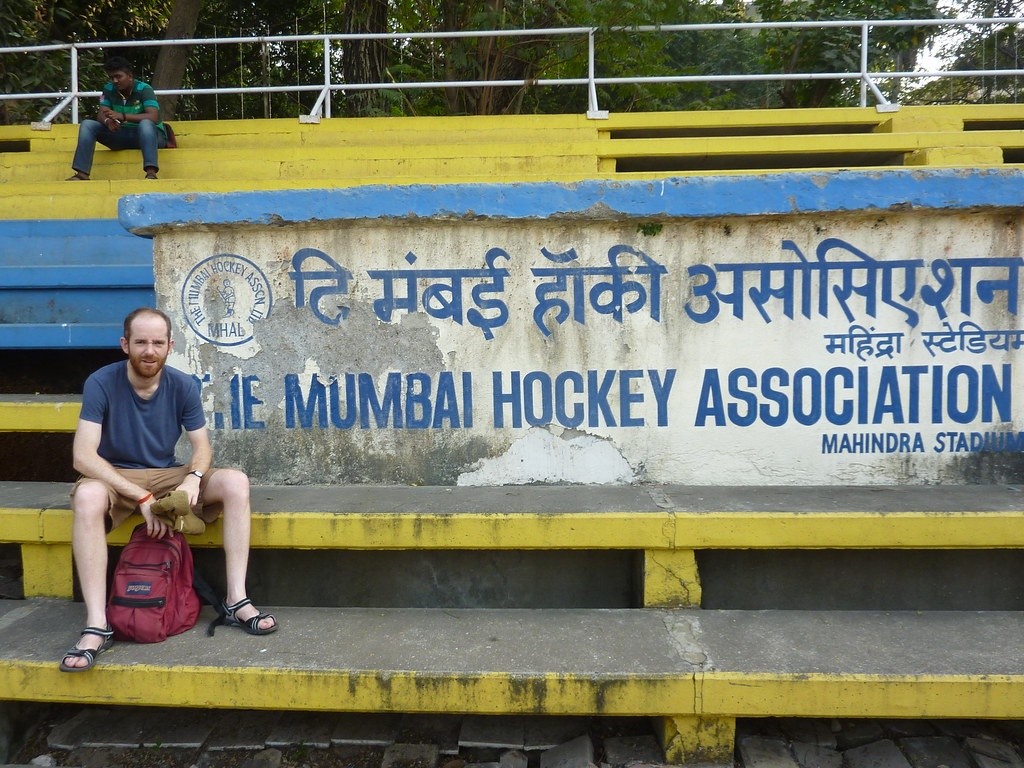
[163,122,177,149]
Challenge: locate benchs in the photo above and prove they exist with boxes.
[0,103,1024,768]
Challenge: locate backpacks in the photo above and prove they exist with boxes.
[106,521,202,644]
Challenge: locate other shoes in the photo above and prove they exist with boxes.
[65,173,90,182]
[145,167,157,180]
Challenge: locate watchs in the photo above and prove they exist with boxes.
[188,470,203,478]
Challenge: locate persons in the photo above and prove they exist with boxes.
[60,308,279,672]
[65,57,167,181]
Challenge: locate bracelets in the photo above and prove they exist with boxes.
[104,118,109,126]
[122,112,126,121]
[138,492,154,504]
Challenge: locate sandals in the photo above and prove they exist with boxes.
[59,624,115,673]
[220,598,279,635]
